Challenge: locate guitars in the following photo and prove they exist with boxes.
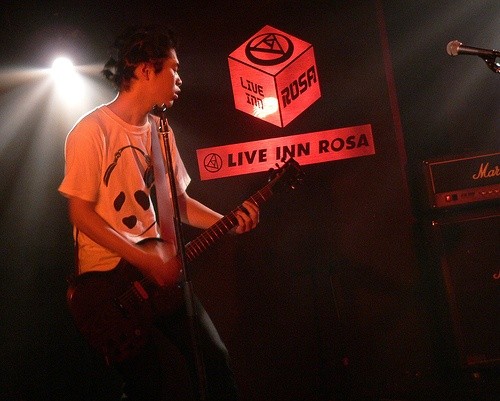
[66,156,304,363]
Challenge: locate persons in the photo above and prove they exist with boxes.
[54,28,266,401]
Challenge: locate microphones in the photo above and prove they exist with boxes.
[447,40,500,58]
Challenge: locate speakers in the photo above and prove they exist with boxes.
[422,207,500,401]
